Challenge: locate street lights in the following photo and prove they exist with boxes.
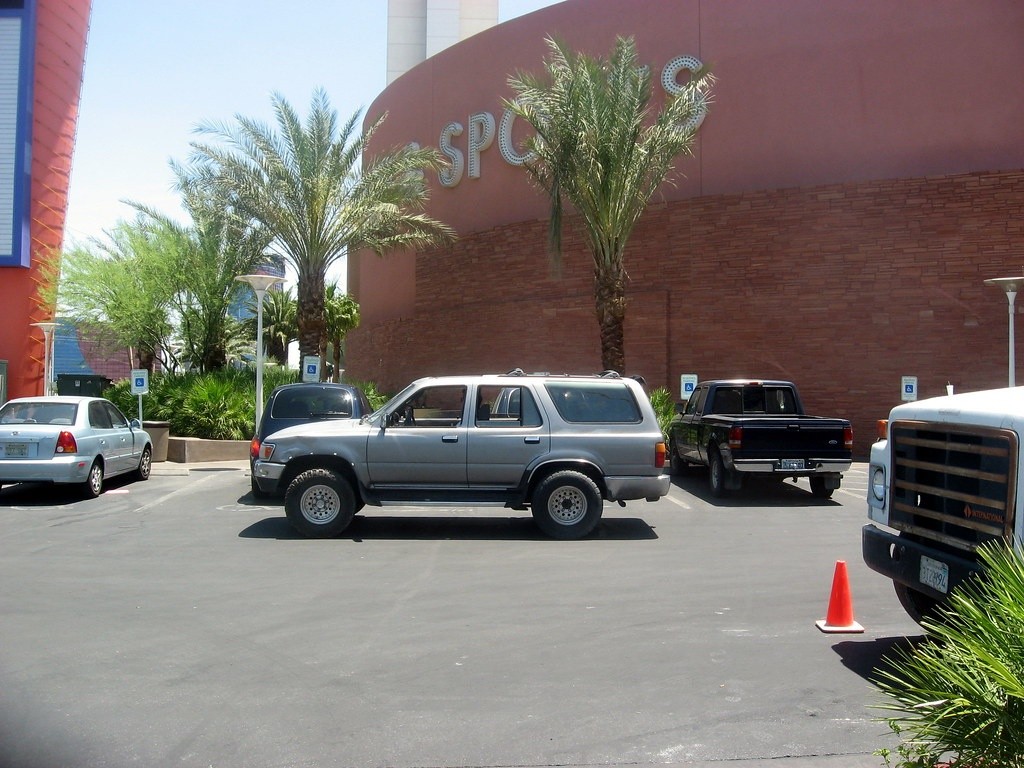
[985,276,1024,387]
[29,322,65,396]
[235,274,288,435]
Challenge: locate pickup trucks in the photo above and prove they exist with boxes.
[670,379,854,500]
[254,367,670,543]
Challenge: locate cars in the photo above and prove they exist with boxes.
[492,388,521,418]
[861,385,1024,633]
[0,396,153,498]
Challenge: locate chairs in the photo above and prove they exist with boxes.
[456,395,482,427]
[763,390,794,413]
[712,391,743,414]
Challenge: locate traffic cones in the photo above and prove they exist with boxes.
[816,560,865,633]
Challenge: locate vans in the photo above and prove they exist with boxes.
[250,382,375,498]
